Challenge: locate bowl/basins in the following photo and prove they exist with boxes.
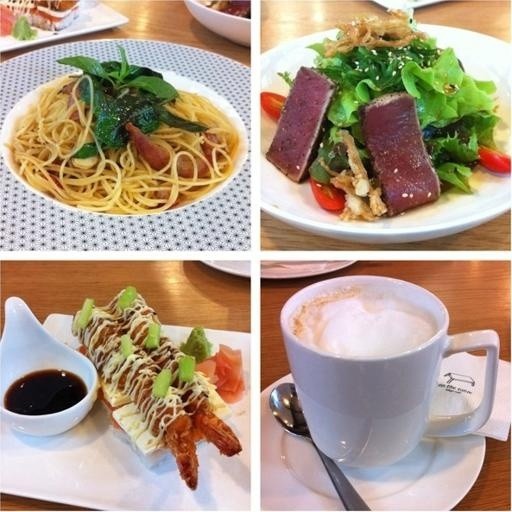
[0,296,99,437]
[183,0,251,47]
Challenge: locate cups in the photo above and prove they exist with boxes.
[280,275,500,472]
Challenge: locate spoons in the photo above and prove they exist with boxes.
[269,383,372,511]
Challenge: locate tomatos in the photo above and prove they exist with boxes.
[310,176,346,210]
[477,144,510,174]
[261,92,287,120]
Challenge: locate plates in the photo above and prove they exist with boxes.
[0,312,251,510]
[260,20,511,244]
[260,371,487,511]
[0,0,130,53]
